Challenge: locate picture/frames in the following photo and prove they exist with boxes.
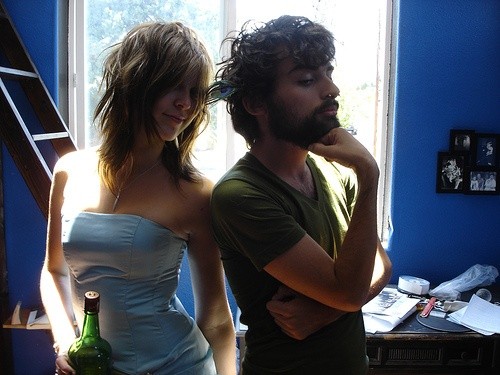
[436,129,500,195]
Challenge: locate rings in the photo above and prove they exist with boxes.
[55,370,58,375]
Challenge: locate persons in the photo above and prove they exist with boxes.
[204,15,392,375]
[470,174,496,191]
[442,163,468,189]
[40,22,236,375]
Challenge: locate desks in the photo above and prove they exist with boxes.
[367,283,500,375]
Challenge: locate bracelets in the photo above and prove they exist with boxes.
[53,321,79,354]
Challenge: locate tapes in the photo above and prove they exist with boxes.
[398,275,429,295]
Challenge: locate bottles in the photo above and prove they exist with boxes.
[67,291,113,375]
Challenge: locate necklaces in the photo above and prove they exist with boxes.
[112,160,161,212]
